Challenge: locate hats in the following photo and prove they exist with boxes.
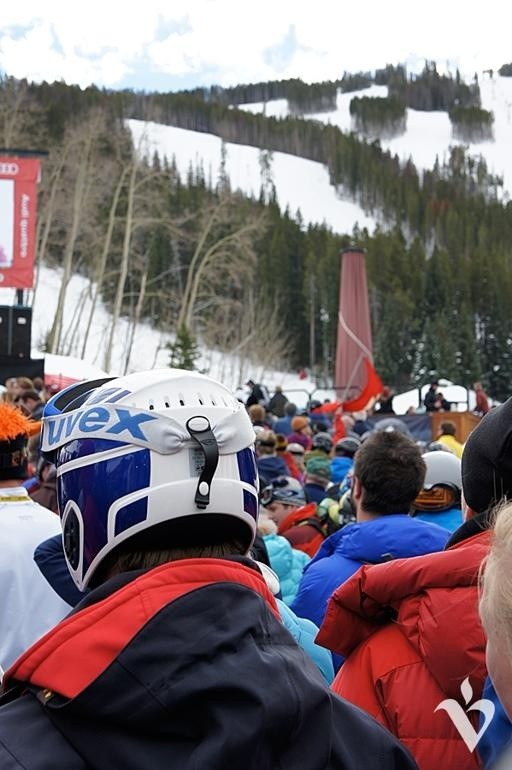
[248,416,512,515]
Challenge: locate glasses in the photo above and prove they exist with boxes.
[40,377,119,464]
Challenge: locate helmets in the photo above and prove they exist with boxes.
[56,368,260,593]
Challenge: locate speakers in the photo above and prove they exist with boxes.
[0,305,32,364]
